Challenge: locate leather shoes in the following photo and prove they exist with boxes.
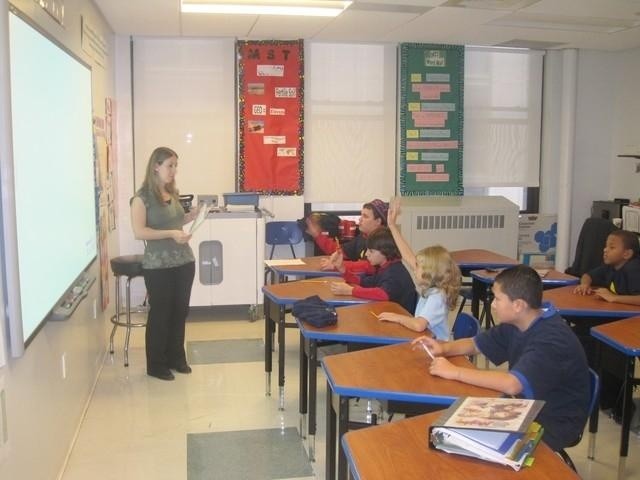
[172,364,192,373]
[147,370,174,381]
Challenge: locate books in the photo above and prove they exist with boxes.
[427,395,546,471]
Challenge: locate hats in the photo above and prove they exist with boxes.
[373,200,389,225]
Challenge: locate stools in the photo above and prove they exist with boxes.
[108,254,152,366]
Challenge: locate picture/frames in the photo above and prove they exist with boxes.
[396,43,466,198]
[235,40,305,198]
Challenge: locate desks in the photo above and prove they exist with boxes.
[447,249,519,312]
[341,408,581,480]
[321,340,506,474]
[260,276,376,386]
[539,283,638,371]
[470,264,580,332]
[262,260,345,351]
[589,315,640,459]
[294,299,437,436]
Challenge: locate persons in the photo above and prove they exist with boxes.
[330,226,417,316]
[377,204,462,341]
[130,146,200,381]
[573,230,640,306]
[304,199,388,270]
[410,265,591,449]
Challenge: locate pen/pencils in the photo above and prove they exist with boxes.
[418,338,436,360]
[369,310,379,318]
[300,280,329,283]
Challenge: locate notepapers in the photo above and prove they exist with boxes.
[407,102,421,111]
[407,163,432,173]
[410,73,422,83]
[411,83,450,100]
[416,173,449,182]
[421,102,455,111]
[412,111,448,127]
[406,152,418,161]
[420,152,449,161]
[406,129,418,139]
[435,164,445,172]
[406,140,458,149]
[420,129,451,137]
[425,72,450,82]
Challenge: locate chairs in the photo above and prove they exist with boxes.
[553,364,598,474]
[447,312,481,371]
[263,222,303,313]
